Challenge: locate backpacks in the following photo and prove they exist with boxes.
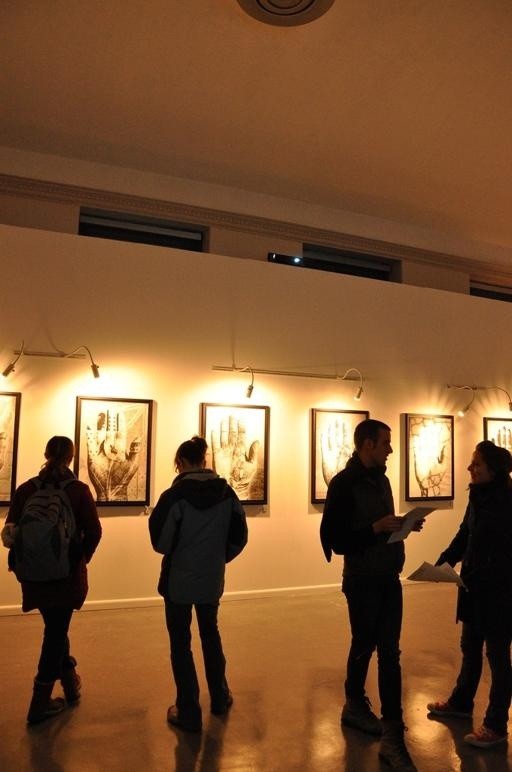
[14,476,81,583]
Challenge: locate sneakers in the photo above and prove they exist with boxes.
[463,724,508,748]
[165,705,203,732]
[427,701,474,719]
[211,689,233,716]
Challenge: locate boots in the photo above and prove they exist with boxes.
[379,717,419,772]
[26,677,66,723]
[60,667,82,702]
[341,680,383,736]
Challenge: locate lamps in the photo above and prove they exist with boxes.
[3,338,105,384]
[445,380,511,418]
[208,359,369,404]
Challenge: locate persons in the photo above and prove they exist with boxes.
[85,407,141,502]
[4,436,102,723]
[491,426,512,454]
[320,419,426,772]
[148,437,248,732]
[208,416,259,500]
[426,440,512,751]
[321,416,356,488]
[414,420,450,498]
[0,431,7,471]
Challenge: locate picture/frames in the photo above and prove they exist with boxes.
[0,389,24,509]
[71,392,155,509]
[307,405,373,507]
[196,399,272,509]
[482,416,512,456]
[402,413,457,503]
